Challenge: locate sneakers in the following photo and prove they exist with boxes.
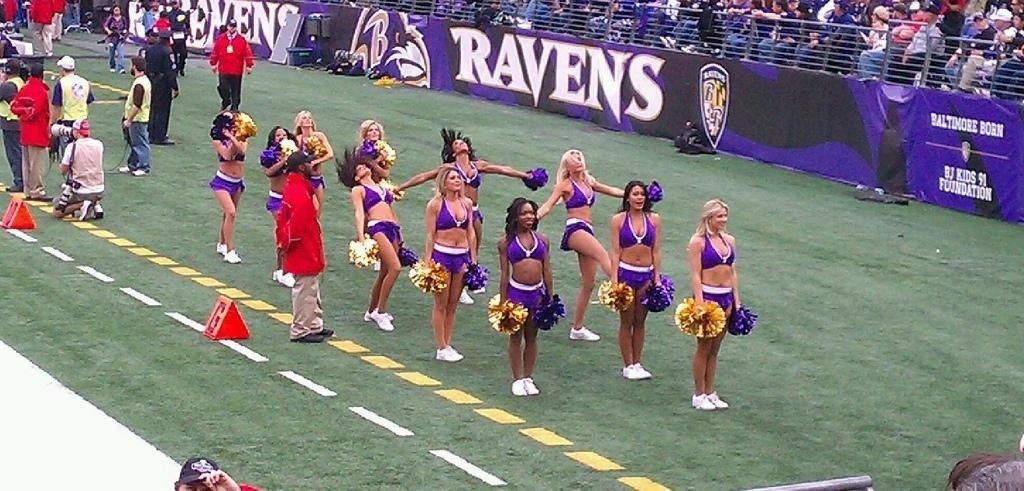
[433,346,464,363]
[119,167,130,173]
[692,390,729,410]
[79,199,104,221]
[6,185,24,192]
[364,310,395,331]
[511,376,539,396]
[272,269,297,288]
[131,169,151,176]
[459,290,474,305]
[313,328,333,336]
[622,363,652,381]
[216,243,227,256]
[568,327,601,342]
[223,250,241,264]
[291,334,324,343]
[157,139,175,145]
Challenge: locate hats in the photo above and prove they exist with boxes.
[179,458,219,483]
[56,56,75,71]
[287,152,314,167]
[73,120,90,136]
[31,63,45,74]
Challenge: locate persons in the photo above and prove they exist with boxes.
[389,130,547,304]
[335,147,402,332]
[422,165,477,363]
[207,110,247,264]
[534,149,740,411]
[257,111,336,288]
[495,197,555,398]
[175,458,257,489]
[1,0,194,222]
[431,0,1024,102]
[210,20,255,108]
[273,150,334,343]
[339,119,404,271]
